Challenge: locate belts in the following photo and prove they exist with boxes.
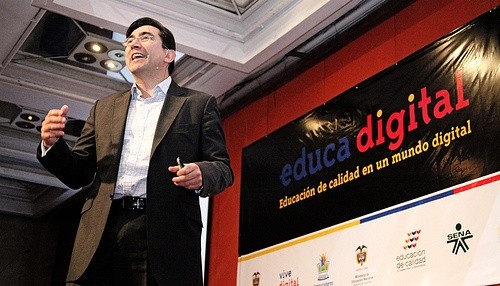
[113,196,148,209]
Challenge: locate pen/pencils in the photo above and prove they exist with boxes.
[175,156,187,168]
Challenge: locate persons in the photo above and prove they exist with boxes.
[37,17,235,286]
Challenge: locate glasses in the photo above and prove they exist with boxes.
[122,35,168,50]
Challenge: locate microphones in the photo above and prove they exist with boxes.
[155,63,169,70]
